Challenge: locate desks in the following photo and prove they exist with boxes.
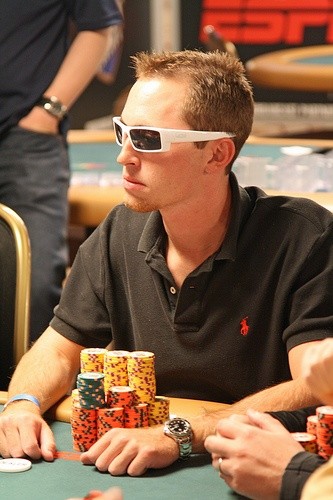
[59,129,332,224]
[247,44,333,91]
[0,390,235,500]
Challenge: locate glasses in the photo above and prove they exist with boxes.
[112,116,238,153]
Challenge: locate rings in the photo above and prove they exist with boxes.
[217,457,223,475]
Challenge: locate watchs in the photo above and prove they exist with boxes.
[162,416,194,461]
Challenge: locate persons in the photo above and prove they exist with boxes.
[0,48,333,477]
[202,332,333,500]
[0,0,129,391]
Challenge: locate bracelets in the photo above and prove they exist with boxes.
[279,451,327,500]
[36,94,68,121]
[0,393,41,413]
